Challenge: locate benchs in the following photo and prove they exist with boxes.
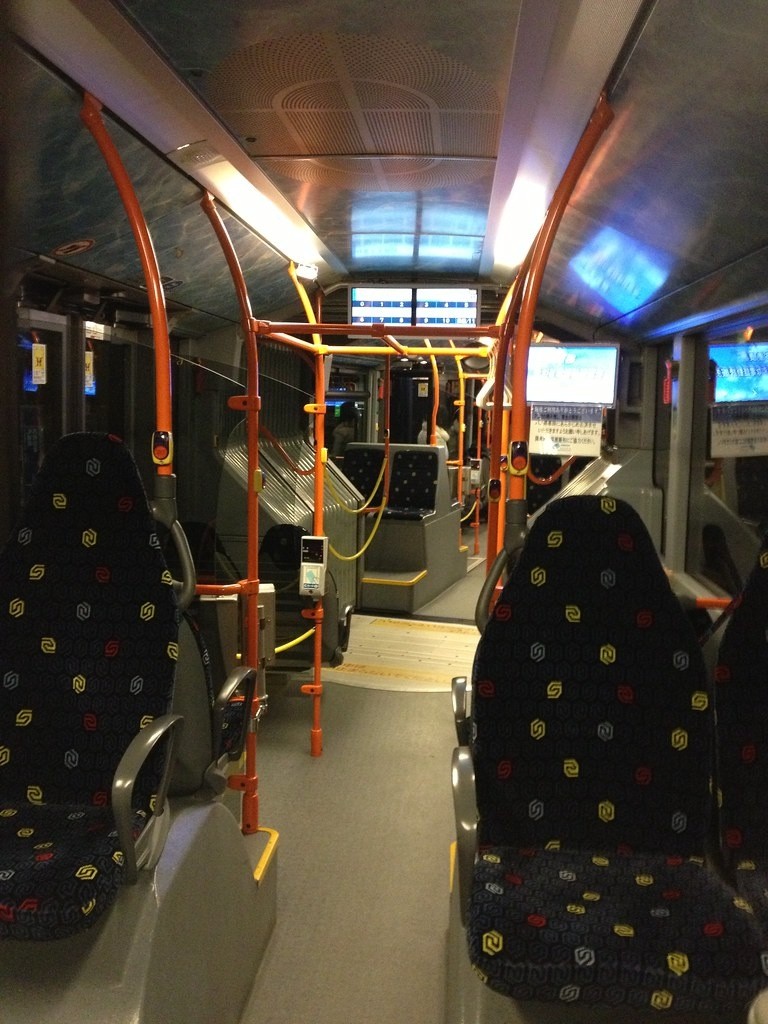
[341,442,451,525]
[445,494,768,1024]
[180,517,313,614]
[0,428,256,939]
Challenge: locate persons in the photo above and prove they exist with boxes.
[332,402,360,454]
[417,410,450,460]
[445,418,459,450]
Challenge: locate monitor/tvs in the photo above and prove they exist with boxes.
[525,344,621,409]
[325,387,348,418]
[347,284,480,340]
[707,342,768,402]
